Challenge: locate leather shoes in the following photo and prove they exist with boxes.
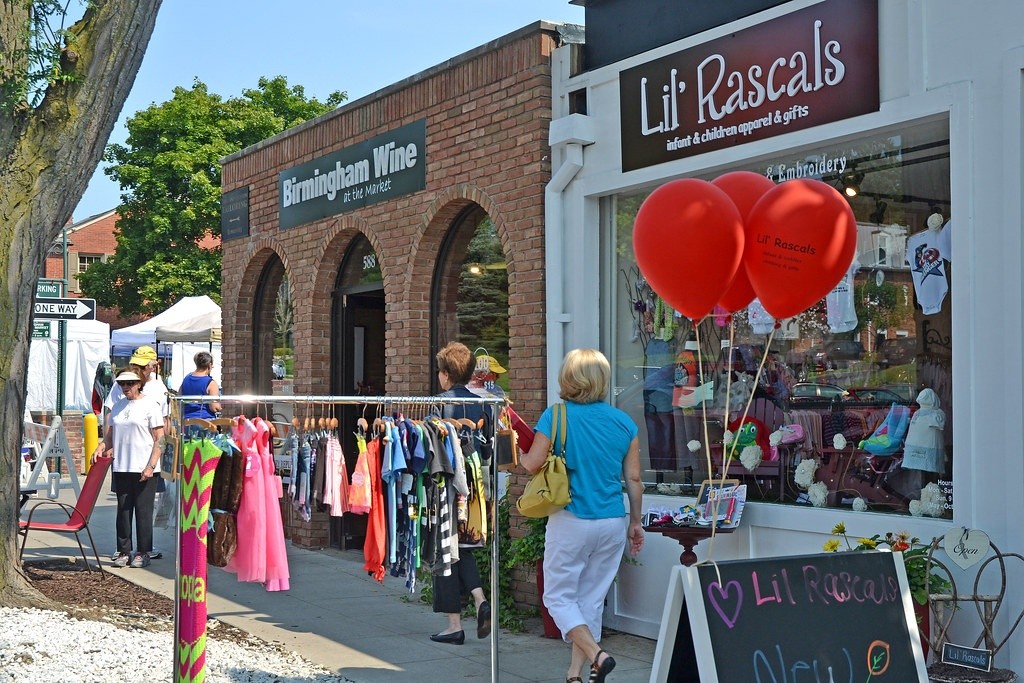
[429,629,465,645]
[477,601,491,638]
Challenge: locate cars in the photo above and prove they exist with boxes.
[806,340,867,370]
[717,345,779,385]
[790,382,919,430]
[876,336,918,370]
[272,363,287,379]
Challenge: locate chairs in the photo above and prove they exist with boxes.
[811,416,917,515]
[19,458,114,581]
[703,390,798,501]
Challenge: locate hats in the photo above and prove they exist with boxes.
[130,346,157,366]
[476,355,507,373]
[114,371,141,380]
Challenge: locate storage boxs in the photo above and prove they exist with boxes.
[497,407,536,454]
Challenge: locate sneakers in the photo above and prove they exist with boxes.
[113,553,130,566]
[131,551,151,567]
[149,546,162,558]
[112,549,122,560]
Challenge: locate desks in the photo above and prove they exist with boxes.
[642,527,737,566]
[20,487,37,508]
[672,407,738,426]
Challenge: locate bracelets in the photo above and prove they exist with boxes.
[147,464,155,469]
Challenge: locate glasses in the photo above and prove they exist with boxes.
[121,380,139,386]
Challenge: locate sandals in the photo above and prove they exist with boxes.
[566,676,582,683]
[588,650,616,683]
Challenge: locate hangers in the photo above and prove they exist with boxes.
[183,396,485,433]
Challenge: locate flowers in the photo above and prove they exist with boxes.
[822,521,963,623]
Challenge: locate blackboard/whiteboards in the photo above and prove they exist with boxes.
[696,478,739,507]
[649,547,930,683]
[160,435,178,481]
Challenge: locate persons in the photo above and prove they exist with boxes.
[643,320,706,484]
[90,345,221,568]
[900,388,945,474]
[519,349,644,683]
[430,341,507,645]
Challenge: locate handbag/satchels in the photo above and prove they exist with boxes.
[516,403,572,517]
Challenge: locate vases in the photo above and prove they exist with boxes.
[912,598,930,665]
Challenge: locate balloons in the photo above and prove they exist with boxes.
[632,170,858,329]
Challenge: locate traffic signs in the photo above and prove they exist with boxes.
[33,297,96,322]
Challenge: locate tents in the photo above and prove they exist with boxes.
[25,294,222,411]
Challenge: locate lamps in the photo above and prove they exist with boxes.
[839,169,866,198]
[924,206,942,228]
[869,198,888,224]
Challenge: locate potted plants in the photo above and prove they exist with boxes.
[800,278,908,366]
[504,514,643,639]
